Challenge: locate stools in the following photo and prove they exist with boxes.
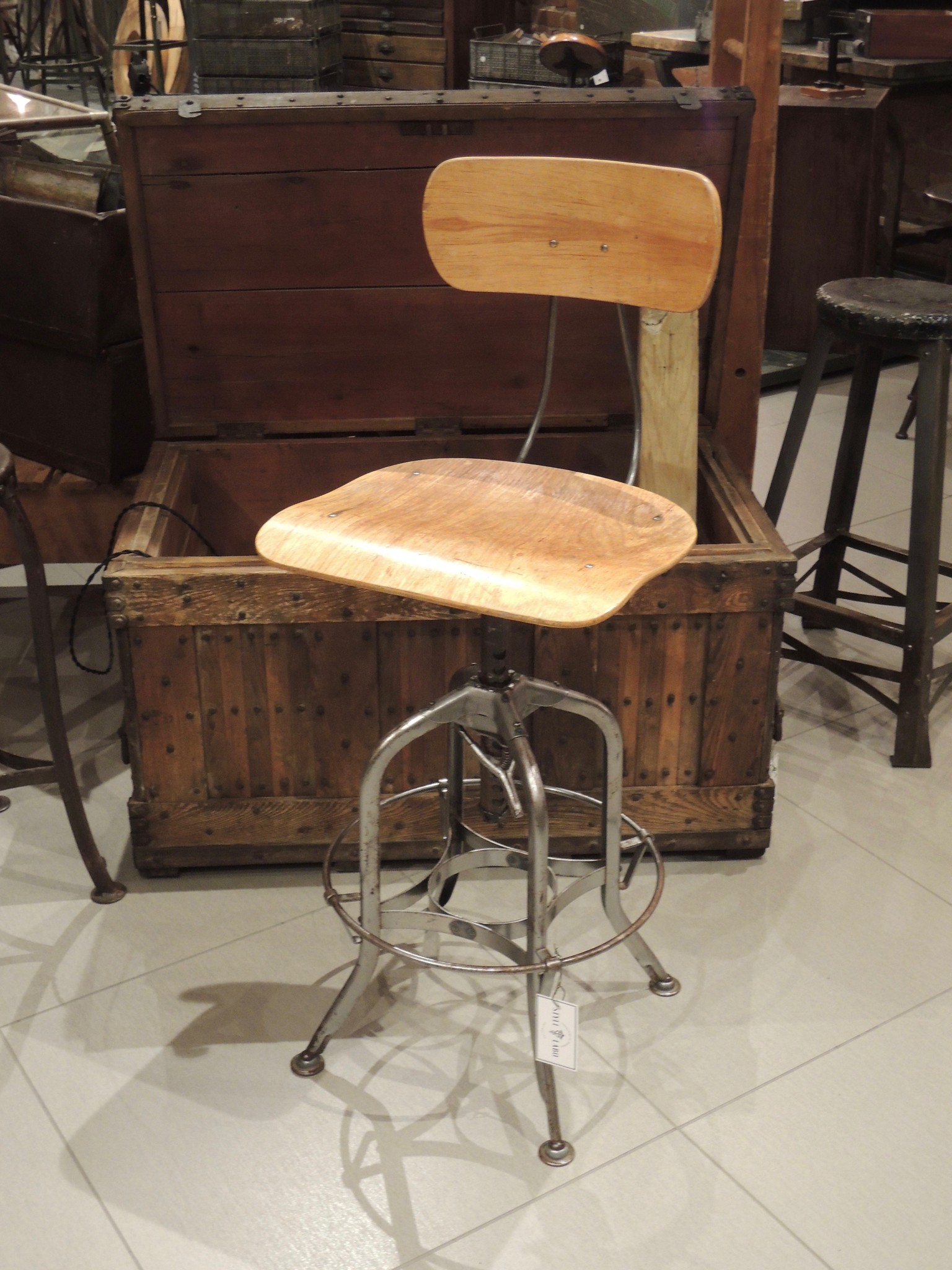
[1,444,132,907]
[761,277,952,768]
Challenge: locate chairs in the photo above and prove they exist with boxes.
[254,157,726,1172]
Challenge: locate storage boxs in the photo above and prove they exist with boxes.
[467,35,626,89]
[856,8,952,57]
[98,87,799,875]
[0,197,162,483]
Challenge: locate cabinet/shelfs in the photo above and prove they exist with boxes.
[629,30,952,390]
[190,0,532,94]
